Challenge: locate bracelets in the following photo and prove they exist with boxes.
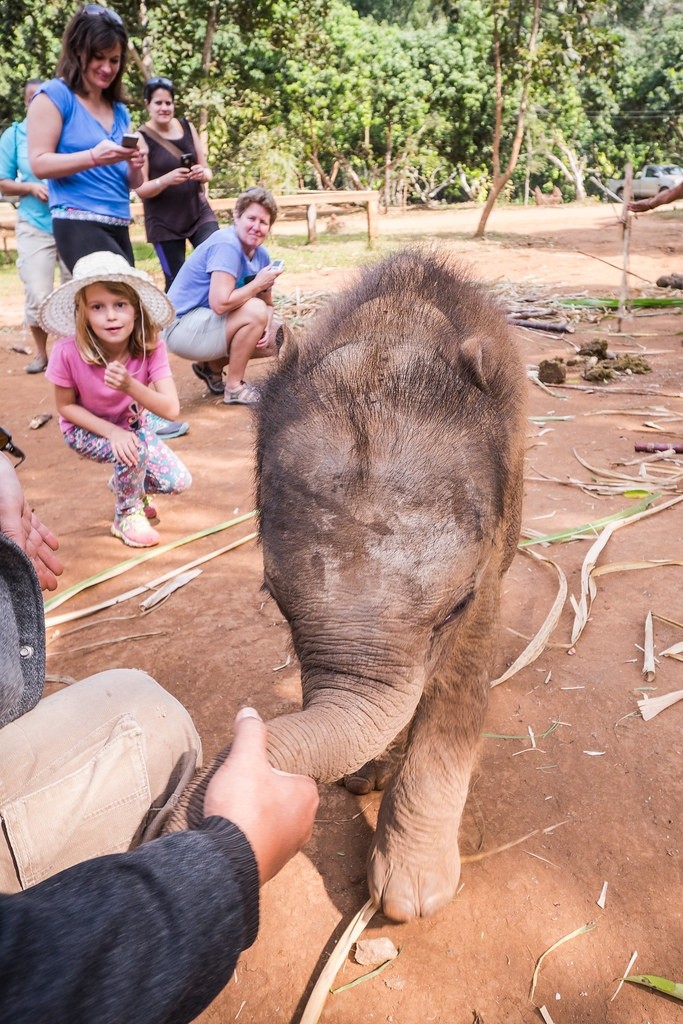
[648,198,654,209]
[90,148,100,167]
[155,178,163,194]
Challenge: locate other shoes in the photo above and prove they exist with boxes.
[24,350,48,373]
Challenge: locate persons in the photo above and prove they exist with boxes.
[36,251,192,547]
[168,186,290,404]
[628,181,683,213]
[27,5,189,439]
[134,77,221,294]
[0,447,320,1024]
[0,78,74,374]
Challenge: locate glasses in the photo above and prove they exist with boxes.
[145,77,174,91]
[78,3,124,26]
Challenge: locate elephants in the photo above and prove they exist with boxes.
[155,246,527,925]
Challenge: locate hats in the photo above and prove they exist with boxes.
[35,251,175,336]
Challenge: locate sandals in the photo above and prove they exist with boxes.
[191,360,225,395]
[224,381,261,404]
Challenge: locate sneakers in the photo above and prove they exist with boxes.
[144,410,191,438]
[110,509,161,547]
[143,493,158,519]
